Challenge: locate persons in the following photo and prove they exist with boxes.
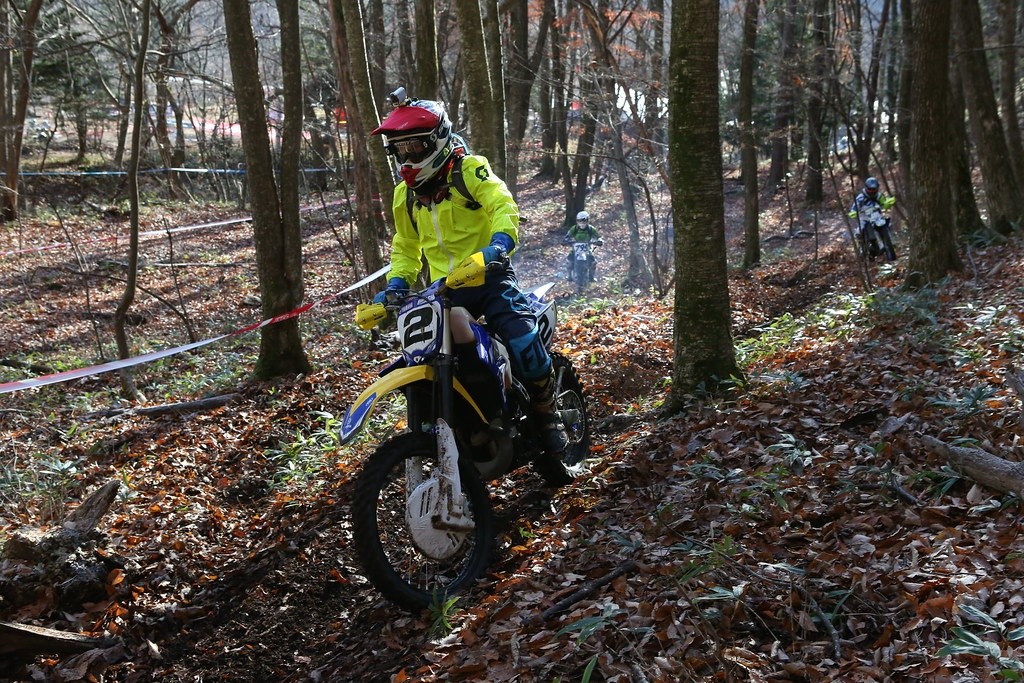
[370,100,568,454]
[850,176,896,255]
[564,211,603,282]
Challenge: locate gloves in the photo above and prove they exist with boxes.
[373,277,410,314]
[480,232,515,274]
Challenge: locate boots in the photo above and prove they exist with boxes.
[589,261,596,282]
[566,260,573,281]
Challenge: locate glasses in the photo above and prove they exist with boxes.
[392,138,435,164]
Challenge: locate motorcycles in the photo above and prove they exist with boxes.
[561,240,602,297]
[848,195,896,261]
[339,251,589,615]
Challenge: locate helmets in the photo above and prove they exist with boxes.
[370,87,470,194]
[862,177,879,201]
[575,211,590,229]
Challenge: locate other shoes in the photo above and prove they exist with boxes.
[529,397,570,454]
